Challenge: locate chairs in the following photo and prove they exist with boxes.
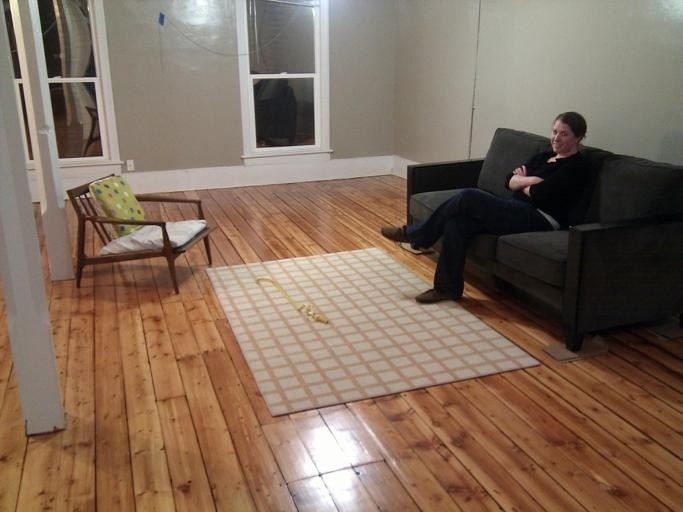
[66,173,217,294]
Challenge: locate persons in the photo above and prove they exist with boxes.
[380,109,586,305]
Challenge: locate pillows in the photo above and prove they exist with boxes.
[599,155,683,224]
[89,175,144,237]
[477,128,553,200]
[539,140,614,225]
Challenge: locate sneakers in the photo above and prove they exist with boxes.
[380,223,414,243]
[415,284,464,304]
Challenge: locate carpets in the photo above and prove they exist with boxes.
[205,247,541,417]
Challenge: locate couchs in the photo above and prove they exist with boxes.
[407,128,683,352]
